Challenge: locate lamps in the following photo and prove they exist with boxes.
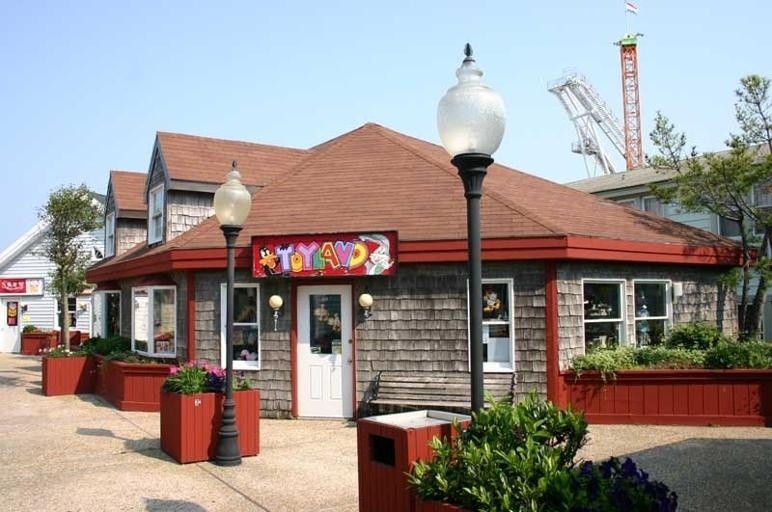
[359,293,373,318]
[22,304,28,311]
[269,295,284,333]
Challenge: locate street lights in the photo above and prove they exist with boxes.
[213,160,252,467]
[438,41,506,422]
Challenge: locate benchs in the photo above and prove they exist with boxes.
[365,368,517,415]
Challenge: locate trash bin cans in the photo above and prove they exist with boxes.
[356,409,473,512]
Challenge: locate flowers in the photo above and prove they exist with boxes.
[37,343,76,357]
[163,357,251,396]
